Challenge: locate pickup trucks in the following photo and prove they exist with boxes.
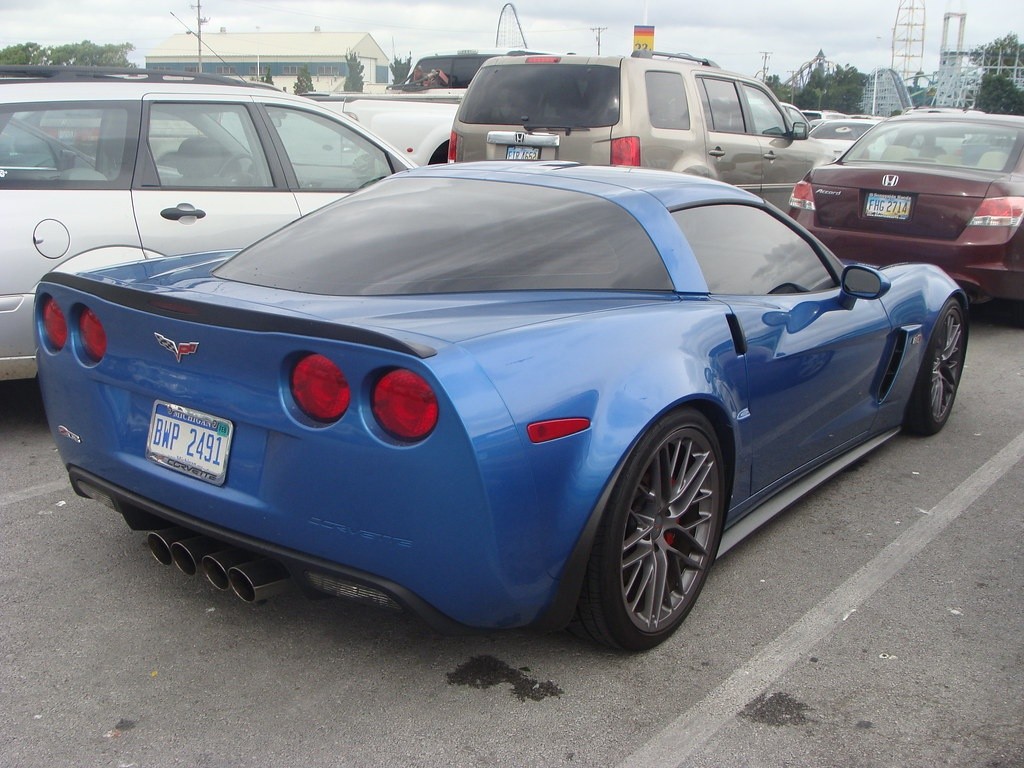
[299,51,509,165]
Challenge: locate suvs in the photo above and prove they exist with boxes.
[0,63,420,380]
[447,49,835,215]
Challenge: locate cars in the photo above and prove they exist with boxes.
[779,102,985,160]
[789,115,1024,305]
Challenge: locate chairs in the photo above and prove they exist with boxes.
[880,145,914,162]
[175,137,236,187]
[978,151,1008,169]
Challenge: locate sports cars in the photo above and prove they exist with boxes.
[33,162,968,655]
